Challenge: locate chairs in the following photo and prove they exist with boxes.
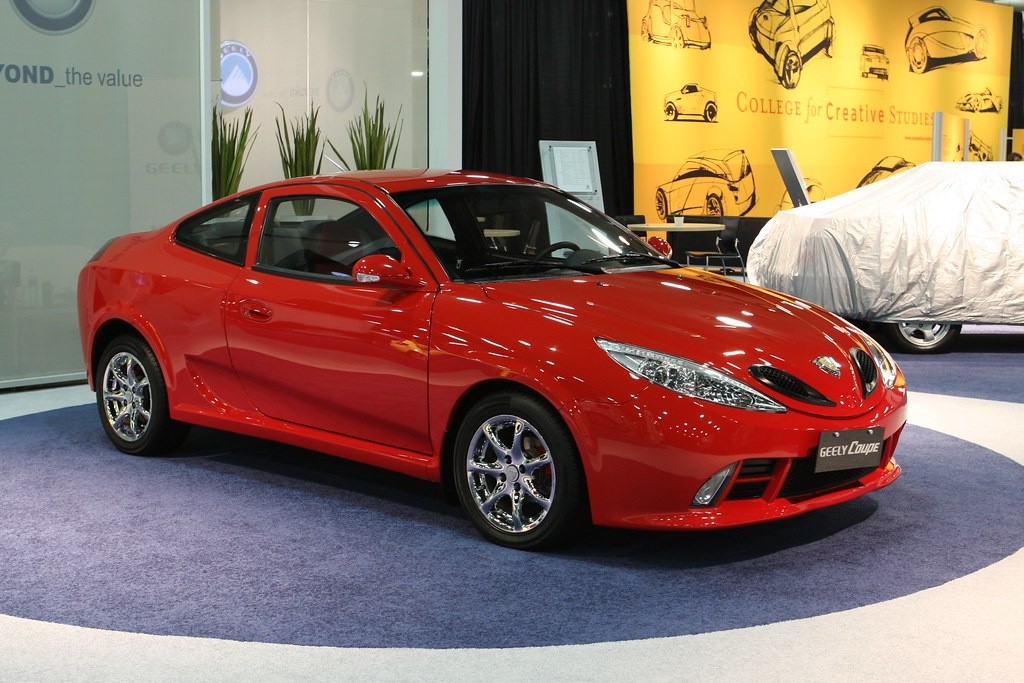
[304,221,384,275]
[686,216,746,283]
[615,215,648,244]
[200,221,246,261]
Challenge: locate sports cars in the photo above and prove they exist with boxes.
[76,170,909,550]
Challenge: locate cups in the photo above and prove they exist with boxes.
[674,217,684,225]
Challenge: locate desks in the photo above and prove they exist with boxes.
[627,224,725,263]
